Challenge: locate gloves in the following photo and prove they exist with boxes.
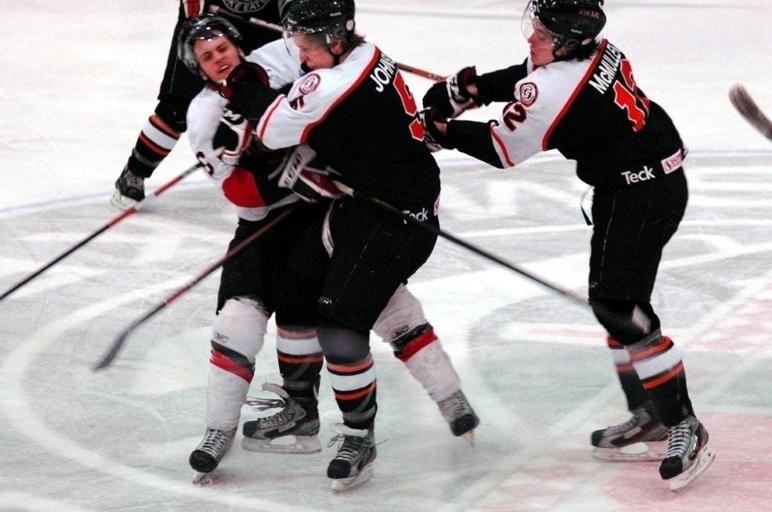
[280,145,343,203]
[225,62,282,129]
[214,111,258,166]
[421,66,481,152]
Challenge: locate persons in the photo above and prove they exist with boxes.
[109,0,284,202]
[223,0,441,480]
[177,14,479,473]
[423,0,715,481]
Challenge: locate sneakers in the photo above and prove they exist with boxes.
[439,391,479,436]
[116,166,147,202]
[189,425,237,473]
[243,375,321,439]
[593,403,666,448]
[327,407,378,479]
[659,414,709,479]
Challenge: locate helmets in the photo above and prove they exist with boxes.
[520,0,607,51]
[175,16,240,76]
[281,0,355,38]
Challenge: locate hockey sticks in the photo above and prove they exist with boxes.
[89,204,298,370]
